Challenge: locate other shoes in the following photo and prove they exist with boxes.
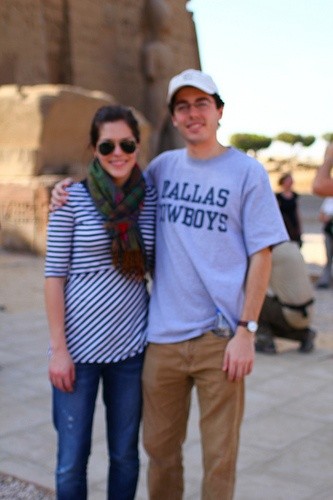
[298,328,315,352]
[255,340,276,354]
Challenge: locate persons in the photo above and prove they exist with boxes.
[311,141,333,288]
[47,68,292,499]
[253,238,316,356]
[275,174,304,250]
[41,105,160,500]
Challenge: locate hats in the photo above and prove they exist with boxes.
[166,69,219,106]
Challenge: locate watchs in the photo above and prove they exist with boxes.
[236,318,259,334]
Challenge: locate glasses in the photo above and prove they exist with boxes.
[96,139,138,156]
